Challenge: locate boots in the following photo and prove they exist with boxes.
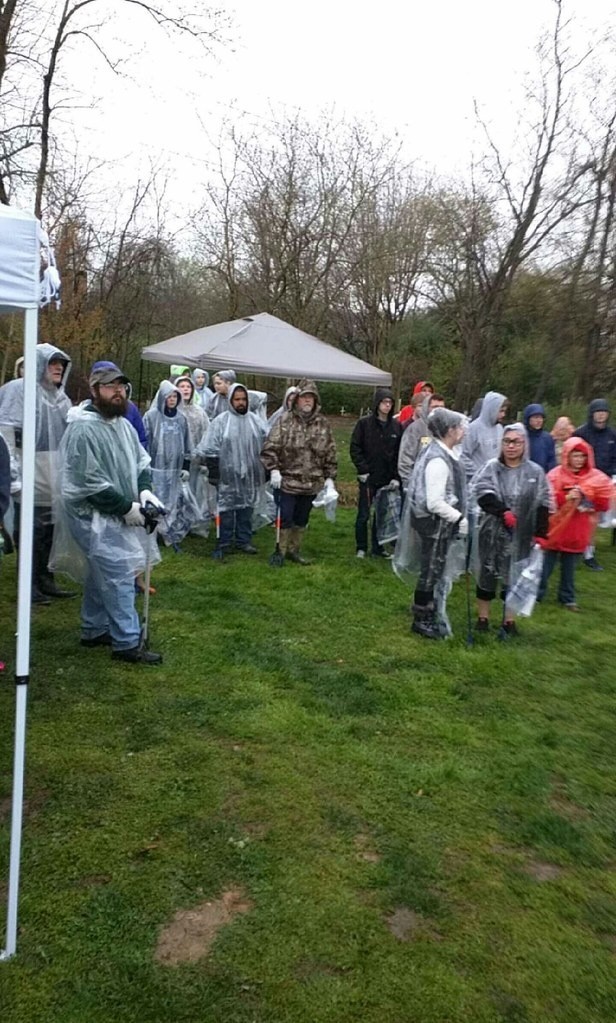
[414,600,445,638]
[14,524,76,603]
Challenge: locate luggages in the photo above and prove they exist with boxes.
[357,550,367,558]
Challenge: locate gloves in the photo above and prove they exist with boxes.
[323,477,334,489]
[389,478,400,490]
[459,517,468,534]
[533,535,549,551]
[200,465,209,476]
[180,469,190,481]
[357,473,370,483]
[504,511,517,528]
[122,501,145,526]
[139,489,165,509]
[270,470,283,489]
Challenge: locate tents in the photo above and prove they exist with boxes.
[138,312,393,409]
[0,201,61,956]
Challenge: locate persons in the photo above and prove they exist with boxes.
[267,386,297,427]
[259,378,338,566]
[470,422,557,635]
[398,395,445,492]
[535,437,616,612]
[349,388,405,561]
[168,365,268,445]
[551,417,575,464]
[392,408,469,637]
[399,382,435,421]
[571,398,616,570]
[402,392,431,429]
[197,383,271,554]
[0,343,78,605]
[459,391,511,575]
[49,367,167,664]
[523,404,557,473]
[141,379,195,547]
[94,361,149,450]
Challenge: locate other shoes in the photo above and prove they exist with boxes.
[505,621,518,636]
[222,546,233,553]
[285,548,310,567]
[475,617,488,630]
[583,557,606,571]
[373,546,394,559]
[136,585,156,594]
[238,542,258,553]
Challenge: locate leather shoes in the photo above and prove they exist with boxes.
[80,630,113,646]
[110,644,163,664]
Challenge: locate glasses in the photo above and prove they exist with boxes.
[381,400,393,404]
[502,437,525,446]
[93,381,129,390]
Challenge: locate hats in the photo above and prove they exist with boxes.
[88,367,132,399]
[49,353,71,362]
